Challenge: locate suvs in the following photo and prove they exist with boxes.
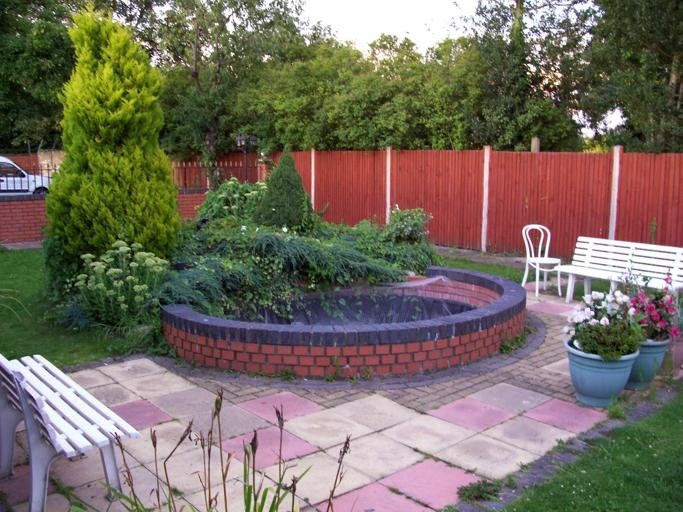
[0,155,51,195]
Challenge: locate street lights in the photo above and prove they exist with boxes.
[235,134,257,184]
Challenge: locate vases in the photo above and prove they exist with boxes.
[625,333,673,391]
[565,335,640,407]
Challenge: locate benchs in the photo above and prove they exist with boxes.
[554,236,683,304]
[0,353,143,512]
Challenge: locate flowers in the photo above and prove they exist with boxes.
[563,290,648,363]
[621,272,681,341]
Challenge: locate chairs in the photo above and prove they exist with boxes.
[521,224,562,296]
